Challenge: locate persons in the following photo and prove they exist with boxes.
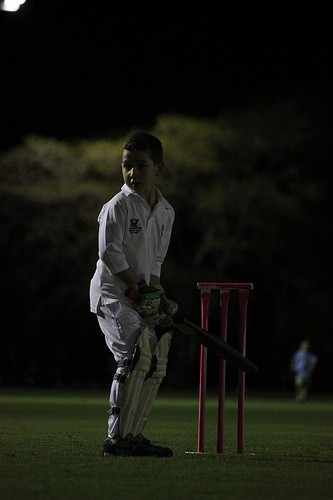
[287,339,319,402]
[88,132,173,457]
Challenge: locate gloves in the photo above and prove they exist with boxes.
[124,279,179,329]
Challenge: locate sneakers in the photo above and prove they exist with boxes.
[101,431,174,458]
